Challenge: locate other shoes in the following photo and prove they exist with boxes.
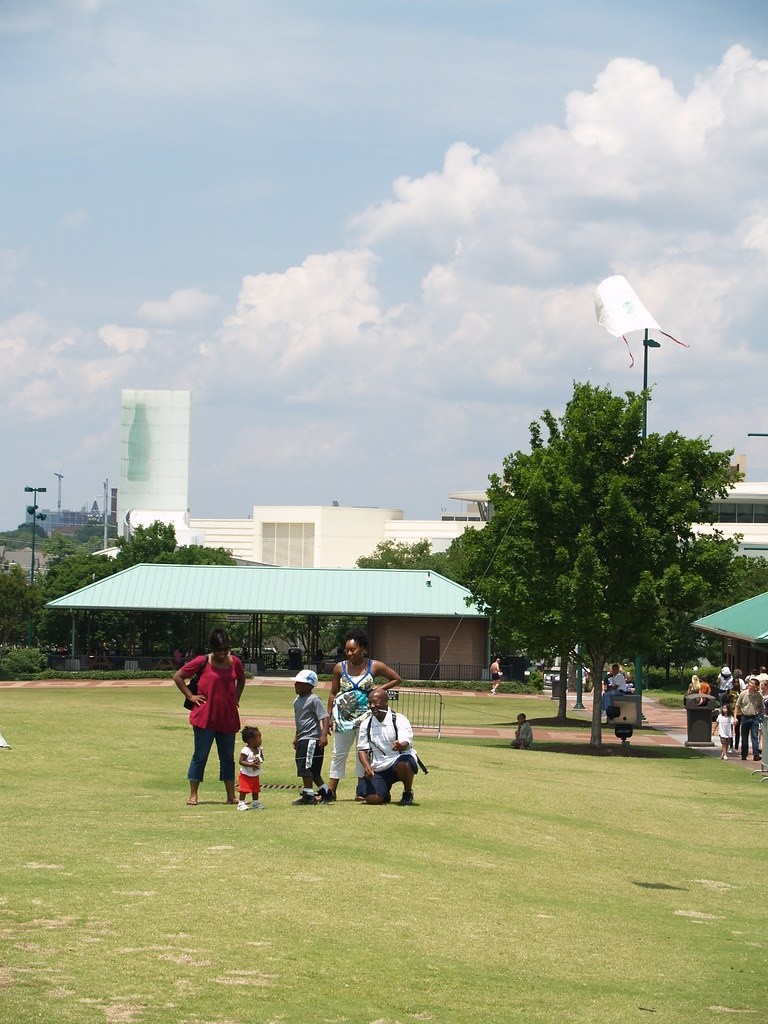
[758,747,762,753]
[382,792,392,803]
[314,788,333,803]
[398,788,415,805]
[251,801,266,809]
[600,710,607,717]
[291,791,318,805]
[720,755,729,761]
[490,689,496,695]
[726,748,740,754]
[741,756,746,760]
[753,755,762,761]
[236,801,250,811]
[355,793,367,801]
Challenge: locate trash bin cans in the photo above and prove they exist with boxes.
[287,647,303,670]
[550,674,560,700]
[682,692,717,741]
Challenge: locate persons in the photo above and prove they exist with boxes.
[599,664,635,716]
[511,713,533,749]
[173,629,245,805]
[291,669,332,805]
[327,630,403,802]
[237,726,265,810]
[687,666,768,761]
[490,658,502,695]
[356,689,418,805]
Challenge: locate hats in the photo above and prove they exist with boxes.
[290,669,318,687]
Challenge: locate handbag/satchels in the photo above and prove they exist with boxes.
[182,655,210,710]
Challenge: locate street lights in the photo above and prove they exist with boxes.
[25,486,46,647]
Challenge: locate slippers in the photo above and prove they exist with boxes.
[186,797,199,805]
[225,797,241,804]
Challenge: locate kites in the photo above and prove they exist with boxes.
[594,275,690,369]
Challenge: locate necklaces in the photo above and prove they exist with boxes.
[351,658,365,667]
